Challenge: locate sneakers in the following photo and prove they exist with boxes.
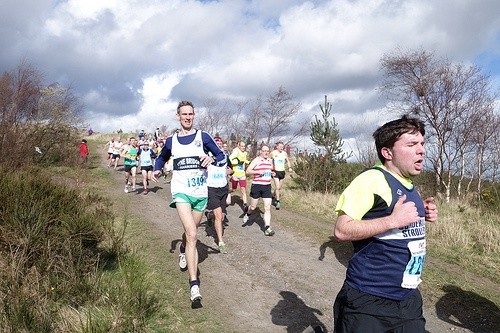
[218,242,227,254]
[265,227,274,236]
[178,252,187,269]
[275,202,280,209]
[243,214,251,223]
[205,211,212,227]
[272,196,276,204]
[190,285,202,302]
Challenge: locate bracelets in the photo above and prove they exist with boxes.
[288,167,291,169]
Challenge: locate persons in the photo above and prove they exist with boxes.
[104,137,168,194]
[150,101,227,304]
[229,142,251,211]
[155,128,159,140]
[299,150,311,158]
[287,145,290,155]
[89,128,93,135]
[334,118,438,333]
[205,137,234,253]
[79,140,89,160]
[244,146,276,235]
[223,143,228,154]
[138,131,144,138]
[271,142,292,209]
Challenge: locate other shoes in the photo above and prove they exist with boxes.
[132,188,136,192]
[125,185,129,192]
[244,206,249,212]
[144,189,148,194]
